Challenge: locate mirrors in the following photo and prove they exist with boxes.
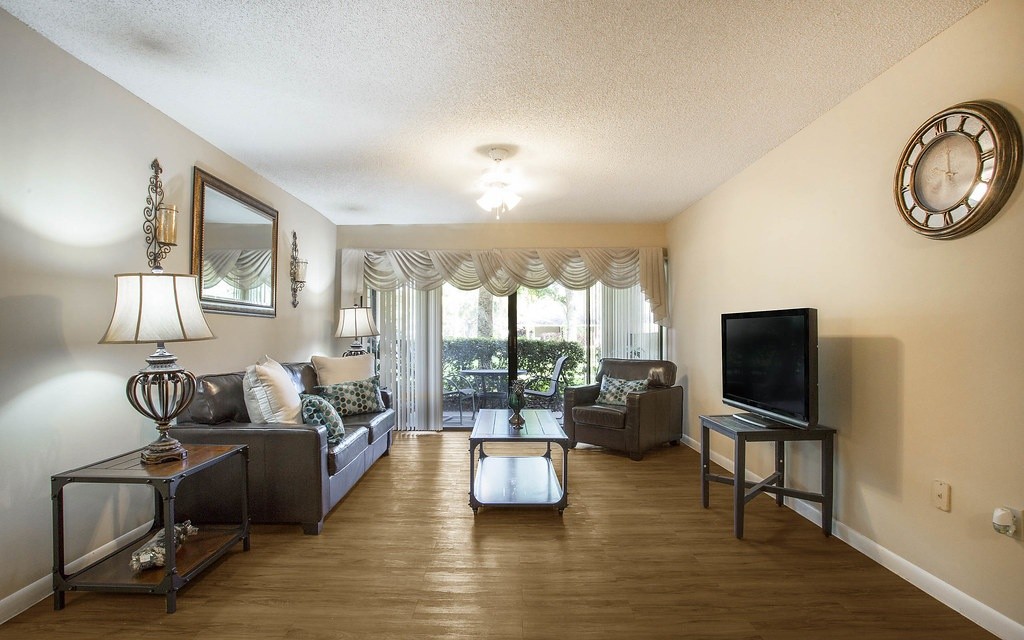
[191,167,279,319]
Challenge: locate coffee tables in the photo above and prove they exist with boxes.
[50,443,250,612]
[469,409,569,514]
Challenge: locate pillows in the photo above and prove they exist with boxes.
[597,373,650,405]
[243,354,386,443]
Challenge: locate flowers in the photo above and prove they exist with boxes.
[509,380,526,393]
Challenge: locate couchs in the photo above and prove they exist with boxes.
[168,363,395,535]
[564,358,684,460]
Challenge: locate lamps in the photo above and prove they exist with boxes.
[334,304,379,356]
[143,161,179,273]
[290,232,308,308]
[477,147,522,218]
[98,274,218,462]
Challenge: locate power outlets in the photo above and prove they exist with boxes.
[933,481,951,512]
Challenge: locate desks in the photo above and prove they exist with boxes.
[462,371,527,419]
[699,414,836,538]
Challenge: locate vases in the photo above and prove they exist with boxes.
[509,392,525,424]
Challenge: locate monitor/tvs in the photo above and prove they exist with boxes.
[720,308,819,430]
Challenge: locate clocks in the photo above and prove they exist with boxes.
[892,99,1022,242]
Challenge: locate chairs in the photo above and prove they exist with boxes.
[443,376,476,425]
[521,356,567,419]
